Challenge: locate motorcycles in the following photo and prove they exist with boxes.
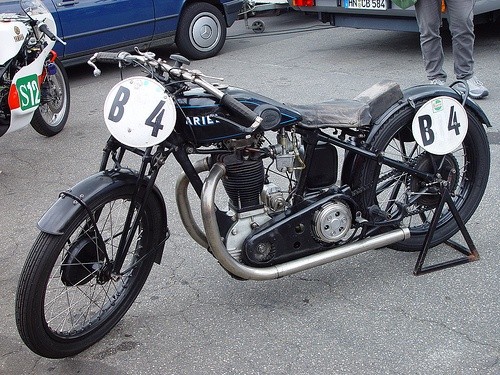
[0,0,70,136]
[14,46,492,359]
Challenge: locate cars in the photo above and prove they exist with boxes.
[0,0,500,68]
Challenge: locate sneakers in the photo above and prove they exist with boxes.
[458,75,488,99]
[430,79,444,87]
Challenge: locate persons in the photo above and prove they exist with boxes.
[414,0,489,99]
